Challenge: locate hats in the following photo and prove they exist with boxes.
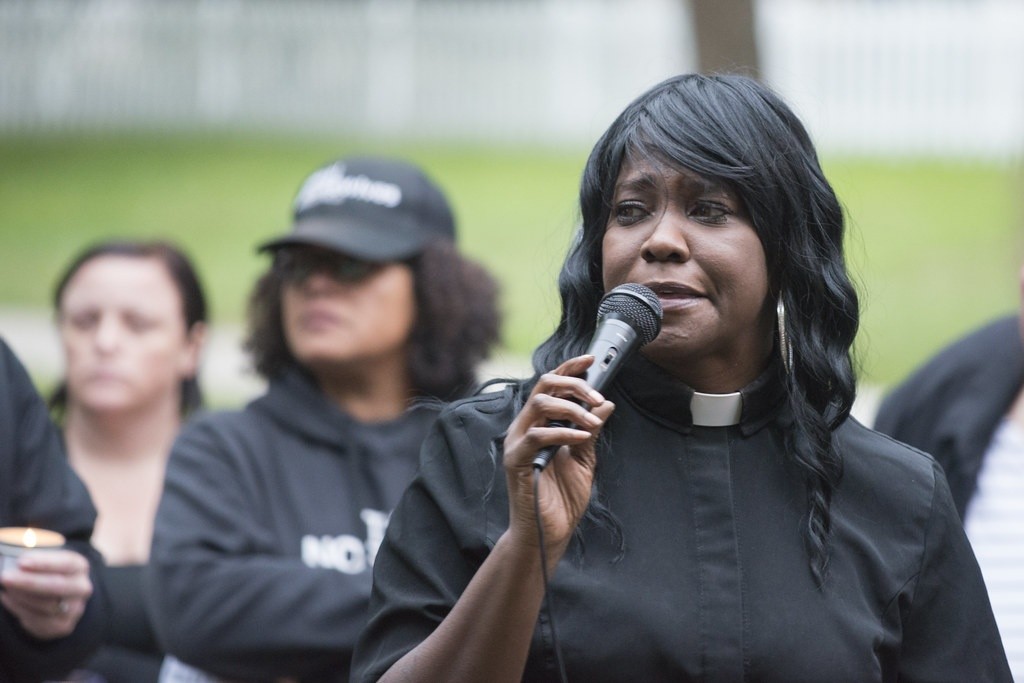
[257,156,454,270]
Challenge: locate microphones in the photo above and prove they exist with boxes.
[531,282,664,473]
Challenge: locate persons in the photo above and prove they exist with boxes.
[47,239,208,683]
[0,338,97,682]
[146,156,497,683]
[351,70,1015,682]
[872,315,1024,682]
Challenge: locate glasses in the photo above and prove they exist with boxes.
[271,246,369,283]
